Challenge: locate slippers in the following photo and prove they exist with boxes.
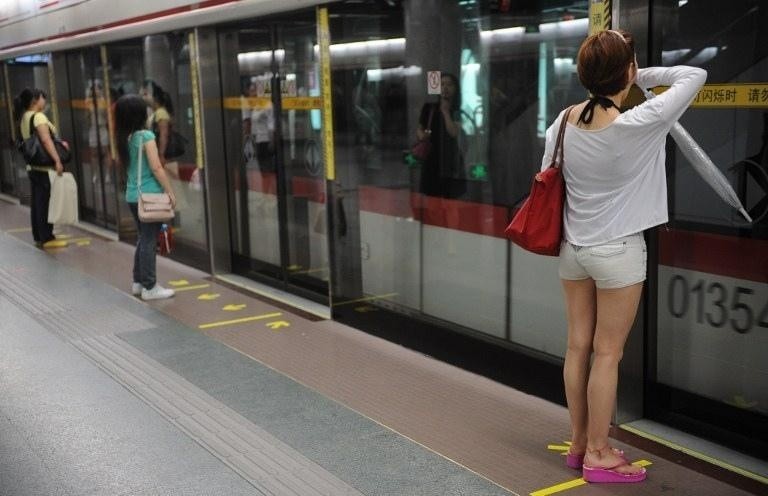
[568,446,648,482]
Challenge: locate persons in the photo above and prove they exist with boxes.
[11,87,70,246]
[86,61,472,235]
[532,27,710,486]
[112,94,178,300]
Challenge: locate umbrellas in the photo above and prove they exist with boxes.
[638,81,752,225]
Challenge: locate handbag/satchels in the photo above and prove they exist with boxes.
[412,130,432,161]
[17,126,73,166]
[46,169,79,226]
[137,193,175,223]
[505,165,565,256]
[153,129,189,158]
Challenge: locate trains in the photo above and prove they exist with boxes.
[3,1,767,458]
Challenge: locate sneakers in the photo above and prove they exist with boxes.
[105,173,111,185]
[132,282,175,300]
[35,235,68,248]
[91,175,99,182]
